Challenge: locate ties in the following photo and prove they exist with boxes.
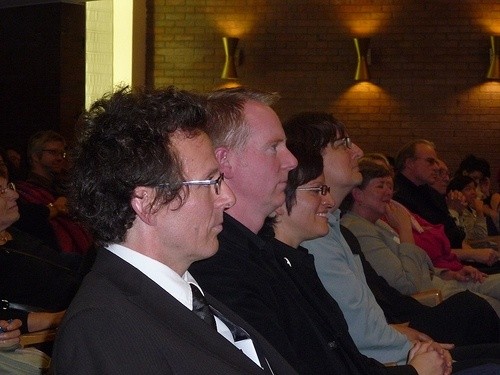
[188,283,250,342]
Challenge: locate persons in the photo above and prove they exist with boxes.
[189,87,500,375]
[0,131,100,345]
[50,85,298,375]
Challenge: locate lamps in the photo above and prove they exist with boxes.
[353,38,372,81]
[220,36,241,80]
[487,35,500,79]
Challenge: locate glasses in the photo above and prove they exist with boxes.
[335,136,352,148]
[41,148,67,158]
[0,182,16,194]
[158,171,225,195]
[296,184,331,196]
